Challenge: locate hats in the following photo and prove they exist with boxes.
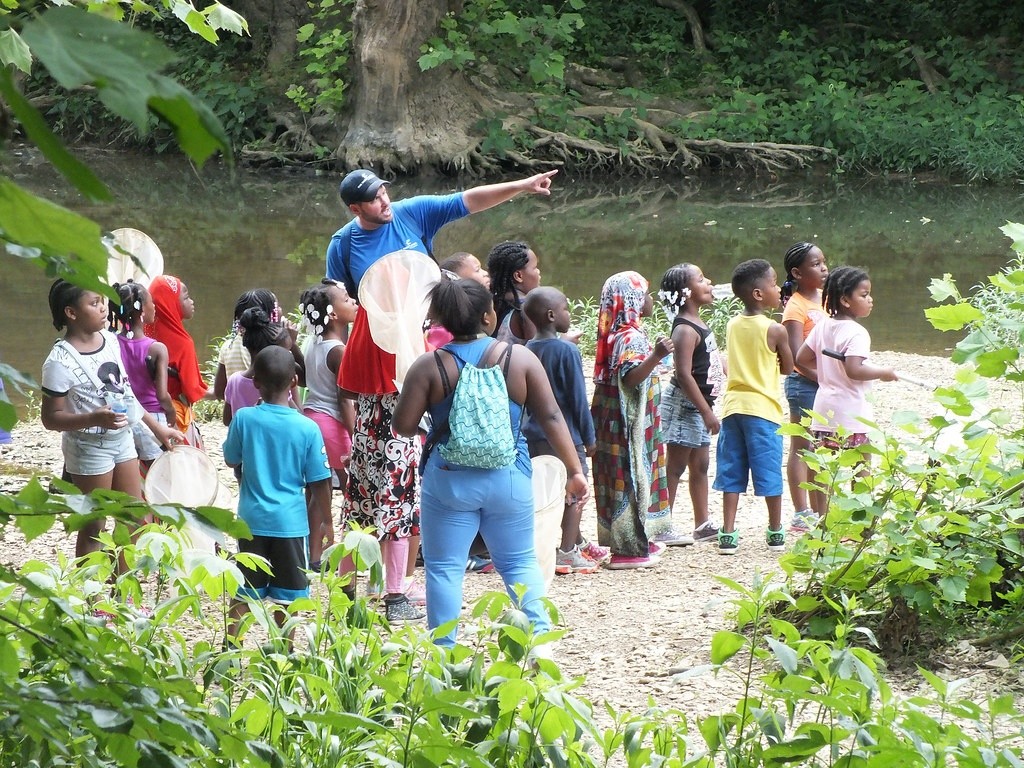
[340,169,392,207]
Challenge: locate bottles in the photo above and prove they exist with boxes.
[110,393,127,414]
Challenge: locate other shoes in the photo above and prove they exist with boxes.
[790,508,820,531]
[307,553,493,627]
[86,595,117,620]
[113,590,156,621]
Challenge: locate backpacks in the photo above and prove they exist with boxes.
[434,341,516,468]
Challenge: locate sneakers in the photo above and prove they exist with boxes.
[555,535,608,573]
[652,529,694,546]
[717,526,739,555]
[692,520,720,542]
[608,540,666,569]
[765,524,786,550]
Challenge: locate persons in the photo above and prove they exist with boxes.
[40,168,900,648]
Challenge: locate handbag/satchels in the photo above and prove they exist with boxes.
[103,381,145,427]
